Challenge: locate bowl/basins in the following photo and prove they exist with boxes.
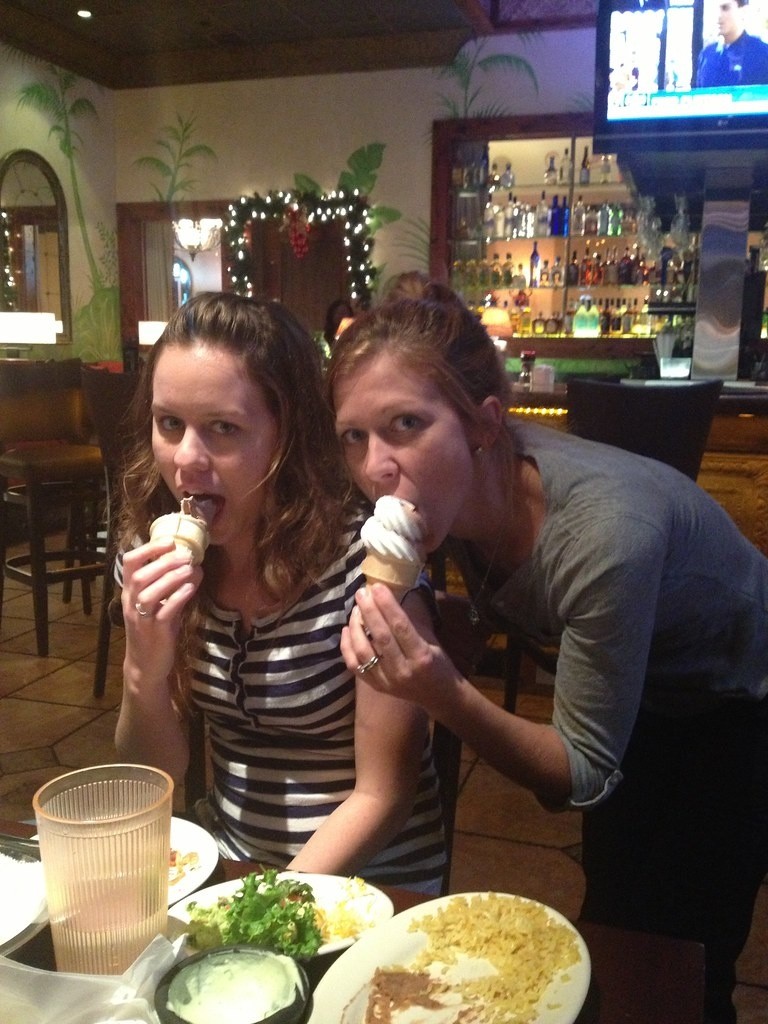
[153,943,309,1024]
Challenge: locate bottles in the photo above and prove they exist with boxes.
[452,146,699,337]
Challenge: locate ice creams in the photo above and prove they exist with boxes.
[150,495,215,571]
[360,495,427,610]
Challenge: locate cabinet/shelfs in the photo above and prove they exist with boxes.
[428,114,768,359]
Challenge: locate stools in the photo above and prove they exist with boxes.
[506,377,721,713]
[0,356,144,700]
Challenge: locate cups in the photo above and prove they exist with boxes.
[33,763,174,975]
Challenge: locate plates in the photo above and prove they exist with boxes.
[167,873,394,954]
[301,891,591,1024]
[170,815,220,905]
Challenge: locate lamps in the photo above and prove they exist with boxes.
[173,219,220,261]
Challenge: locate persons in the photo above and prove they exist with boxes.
[323,298,353,345]
[327,272,768,1024]
[691,0,768,86]
[106,290,446,894]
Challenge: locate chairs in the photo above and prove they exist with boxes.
[432,589,474,898]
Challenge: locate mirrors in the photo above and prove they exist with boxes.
[0,149,73,345]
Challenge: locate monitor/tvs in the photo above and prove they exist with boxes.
[594,0,768,153]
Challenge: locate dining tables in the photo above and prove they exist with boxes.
[4,857,707,1024]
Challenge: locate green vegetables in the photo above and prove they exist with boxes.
[225,867,323,965]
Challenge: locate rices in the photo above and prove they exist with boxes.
[312,876,381,940]
[402,890,580,1019]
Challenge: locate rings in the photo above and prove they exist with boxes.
[135,603,146,615]
[357,657,378,674]
